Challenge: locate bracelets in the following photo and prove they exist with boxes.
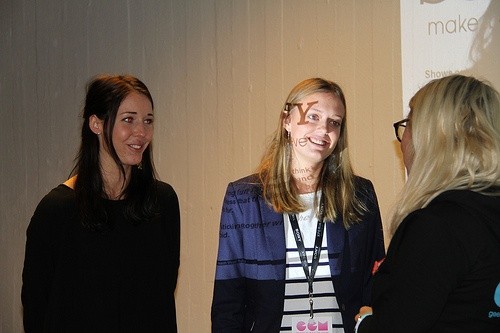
[357,312,372,321]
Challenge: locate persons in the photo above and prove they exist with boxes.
[354,75,499,333]
[210,78,385,333]
[19,73,180,333]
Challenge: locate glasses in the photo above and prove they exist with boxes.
[393,119,410,143]
